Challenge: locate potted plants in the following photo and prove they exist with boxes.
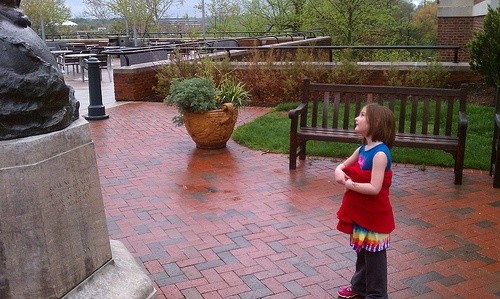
[162,73,252,149]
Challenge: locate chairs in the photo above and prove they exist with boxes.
[489,86,500,187]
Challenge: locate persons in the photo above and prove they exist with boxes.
[337,103,395,299]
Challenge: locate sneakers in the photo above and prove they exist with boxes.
[337,285,365,298]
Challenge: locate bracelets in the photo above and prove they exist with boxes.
[352,182,358,191]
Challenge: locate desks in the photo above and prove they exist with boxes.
[48,41,128,82]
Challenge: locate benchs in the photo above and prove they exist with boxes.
[287,78,471,184]
